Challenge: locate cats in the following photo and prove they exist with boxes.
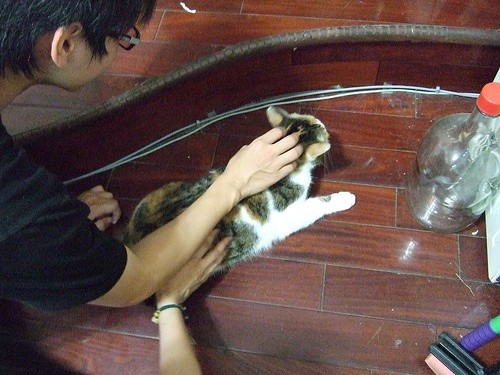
[121,104,356,307]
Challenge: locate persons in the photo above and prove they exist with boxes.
[155,228,232,375]
[0,0,304,312]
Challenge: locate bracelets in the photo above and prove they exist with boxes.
[151,304,187,326]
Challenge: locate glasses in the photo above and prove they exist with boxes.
[113,25,141,51]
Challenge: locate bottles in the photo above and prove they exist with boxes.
[406,82,499,233]
